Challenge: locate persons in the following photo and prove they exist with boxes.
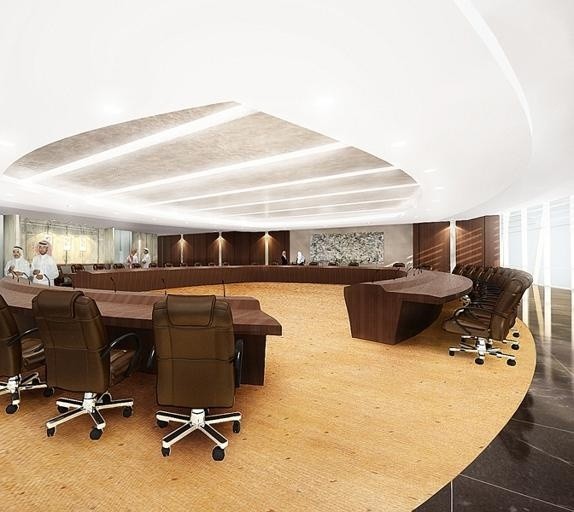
[4,245,30,278]
[127,248,139,268]
[30,242,59,286]
[142,248,151,268]
[296,251,304,264]
[281,250,288,264]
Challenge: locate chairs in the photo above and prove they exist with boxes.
[71,262,142,273]
[149,260,231,268]
[449,262,535,365]
[0,294,54,415]
[32,291,141,441]
[146,294,241,461]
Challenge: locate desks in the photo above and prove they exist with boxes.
[0,286,283,388]
[385,270,474,345]
